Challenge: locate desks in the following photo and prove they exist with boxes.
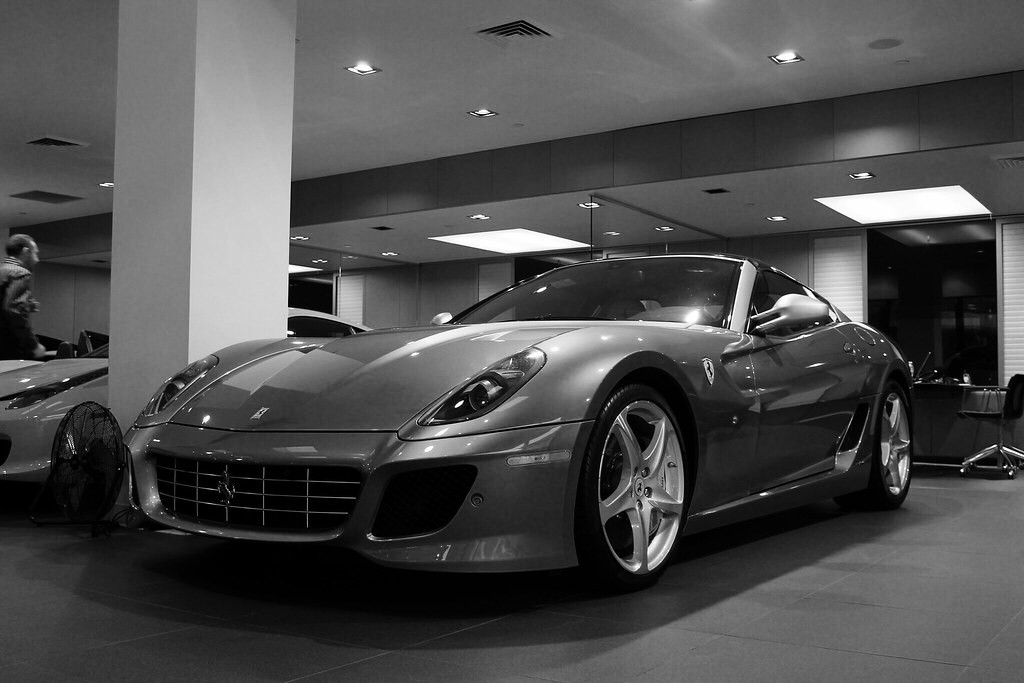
[913,383,976,468]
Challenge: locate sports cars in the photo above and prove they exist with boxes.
[120,251,917,598]
[0,303,378,485]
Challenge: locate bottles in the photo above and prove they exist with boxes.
[908,361,914,377]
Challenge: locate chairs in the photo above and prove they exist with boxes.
[957,373,1023,476]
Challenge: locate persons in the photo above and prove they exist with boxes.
[0,234,46,361]
